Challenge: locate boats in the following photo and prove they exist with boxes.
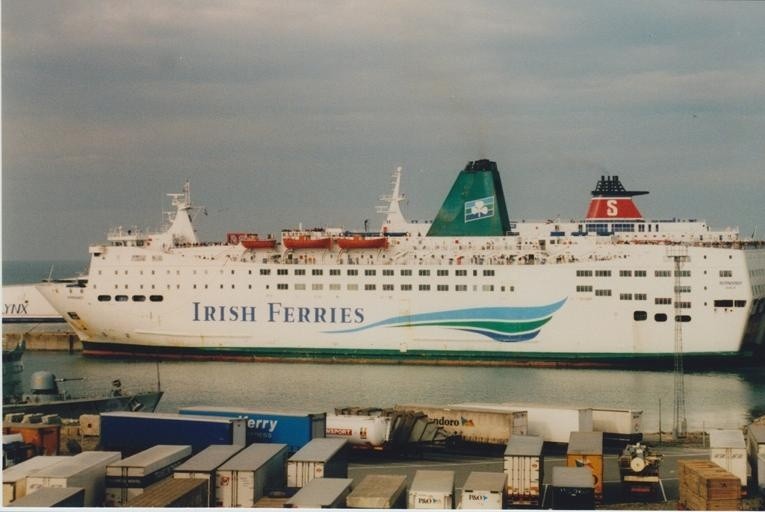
[239,236,276,248]
[336,233,387,250]
[281,235,331,249]
[2,275,88,323]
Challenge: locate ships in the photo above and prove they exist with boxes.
[29,152,764,370]
[2,332,167,424]
